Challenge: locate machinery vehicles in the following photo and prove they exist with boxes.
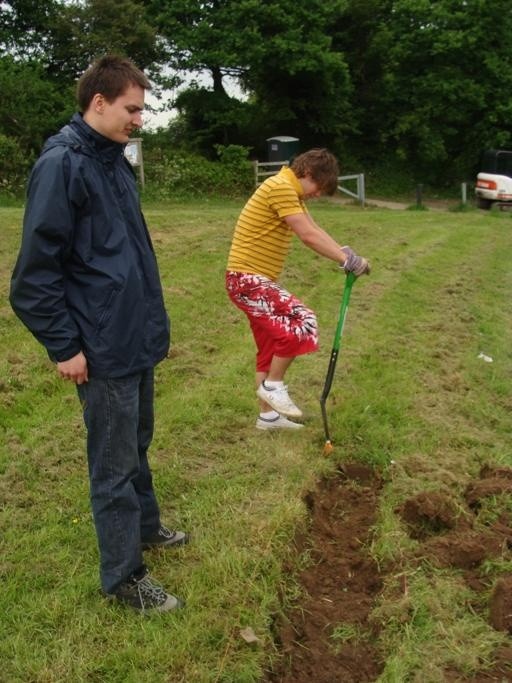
[474,149,512,211]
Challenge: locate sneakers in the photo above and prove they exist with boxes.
[147,525,188,547]
[115,575,179,616]
[256,379,304,431]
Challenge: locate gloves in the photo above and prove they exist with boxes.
[340,246,370,276]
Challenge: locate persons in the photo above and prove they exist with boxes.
[9,54,188,617]
[226,148,371,430]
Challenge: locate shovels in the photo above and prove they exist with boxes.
[320,271,356,441]
